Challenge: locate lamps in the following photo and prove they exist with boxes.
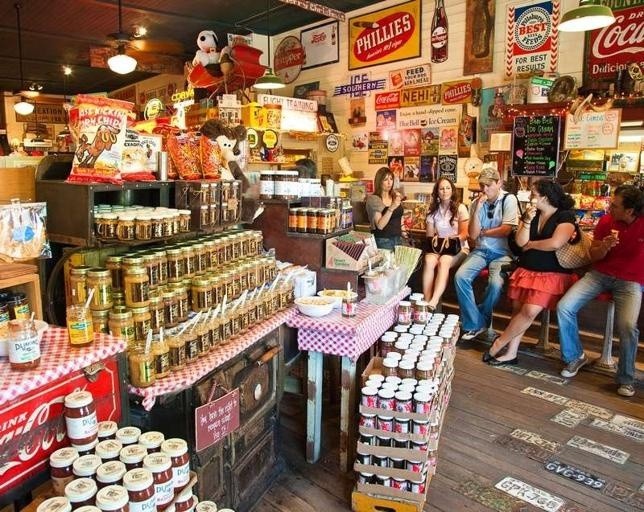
[108,0,136,74]
[37,85,43,90]
[12,4,34,117]
[29,82,35,90]
[557,0,615,32]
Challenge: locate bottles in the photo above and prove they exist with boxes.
[237,307,248,336]
[196,499,217,512]
[168,336,186,372]
[152,216,163,238]
[0,299,9,321]
[115,426,141,445]
[392,438,409,449]
[179,210,191,232]
[409,323,425,335]
[70,264,92,307]
[197,325,211,359]
[411,418,429,437]
[121,468,157,512]
[364,379,381,388]
[413,392,431,415]
[409,342,424,351]
[209,204,217,226]
[122,254,150,307]
[172,213,178,234]
[306,208,317,234]
[232,273,240,299]
[358,472,374,485]
[402,354,418,363]
[286,170,299,200]
[86,267,113,311]
[271,292,278,315]
[209,275,222,309]
[438,314,459,344]
[376,389,394,411]
[231,203,238,222]
[137,431,164,453]
[162,216,173,237]
[381,382,398,392]
[129,307,152,344]
[72,453,102,480]
[6,318,41,369]
[240,267,247,292]
[385,351,401,361]
[63,390,99,446]
[136,215,152,240]
[108,311,135,344]
[221,202,228,224]
[94,484,129,512]
[102,215,117,239]
[393,324,409,334]
[425,335,443,354]
[222,229,264,266]
[400,332,414,340]
[161,438,191,493]
[6,292,30,321]
[183,330,198,364]
[218,317,231,345]
[397,383,415,392]
[341,292,358,318]
[96,419,117,440]
[247,305,257,330]
[422,312,445,336]
[35,496,72,512]
[181,245,194,279]
[73,505,100,512]
[172,283,188,322]
[397,360,415,378]
[328,198,354,235]
[397,301,411,325]
[408,294,420,318]
[359,387,377,408]
[405,459,422,473]
[140,251,158,290]
[262,293,272,319]
[175,490,194,511]
[258,170,274,200]
[358,412,377,431]
[49,447,79,497]
[394,391,412,413]
[375,476,390,487]
[274,170,288,200]
[296,208,307,233]
[381,358,398,376]
[418,379,434,389]
[150,340,170,379]
[210,182,217,208]
[206,319,221,352]
[106,254,125,294]
[422,349,435,357]
[374,435,392,448]
[148,291,164,334]
[228,312,241,340]
[64,477,98,508]
[191,277,212,313]
[95,438,122,461]
[380,335,394,358]
[214,238,223,268]
[161,289,178,328]
[412,292,424,301]
[407,476,425,494]
[371,454,388,467]
[390,477,408,492]
[200,206,209,226]
[245,253,277,290]
[118,444,151,473]
[417,355,434,363]
[376,415,394,433]
[403,348,419,356]
[410,439,426,452]
[192,243,206,276]
[393,414,411,434]
[385,374,400,385]
[204,240,216,272]
[92,310,108,334]
[414,334,428,342]
[384,330,398,339]
[288,207,297,233]
[415,361,432,379]
[143,453,177,512]
[358,432,375,447]
[93,204,180,218]
[423,312,433,319]
[231,181,240,200]
[166,248,183,283]
[412,301,428,324]
[66,302,94,348]
[317,211,331,235]
[222,279,233,304]
[254,299,264,325]
[414,385,431,394]
[95,460,126,486]
[201,183,209,209]
[118,216,134,241]
[155,250,168,286]
[401,377,417,386]
[355,453,370,465]
[128,346,155,387]
[368,374,384,382]
[92,213,104,237]
[388,457,405,470]
[277,284,295,312]
[223,182,231,201]
[394,341,409,352]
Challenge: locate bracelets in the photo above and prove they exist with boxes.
[522,218,532,231]
[400,223,405,226]
[388,208,392,212]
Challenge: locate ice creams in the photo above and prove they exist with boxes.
[530,198,537,206]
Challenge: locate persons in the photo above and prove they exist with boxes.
[561,184,642,396]
[453,169,518,342]
[421,177,469,310]
[483,179,580,366]
[366,168,405,252]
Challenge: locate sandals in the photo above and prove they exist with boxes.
[488,356,518,366]
[483,336,502,362]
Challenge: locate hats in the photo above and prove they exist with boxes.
[478,168,499,185]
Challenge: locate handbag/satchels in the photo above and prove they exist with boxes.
[502,193,525,255]
[555,220,595,269]
[426,236,460,255]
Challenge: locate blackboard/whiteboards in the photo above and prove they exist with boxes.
[511,115,561,179]
[563,109,621,151]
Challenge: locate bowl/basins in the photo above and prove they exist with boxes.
[294,296,335,316]
[318,290,357,301]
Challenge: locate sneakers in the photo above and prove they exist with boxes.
[462,329,487,340]
[560,354,588,377]
[617,384,635,396]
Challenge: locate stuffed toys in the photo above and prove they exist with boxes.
[203,119,246,177]
[191,30,220,68]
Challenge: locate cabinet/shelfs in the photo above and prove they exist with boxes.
[47,247,299,512]
[246,197,390,301]
[35,177,243,248]
[1,263,44,320]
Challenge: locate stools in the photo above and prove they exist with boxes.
[573,270,617,371]
[480,269,498,341]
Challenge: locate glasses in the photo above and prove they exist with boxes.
[487,203,495,219]
